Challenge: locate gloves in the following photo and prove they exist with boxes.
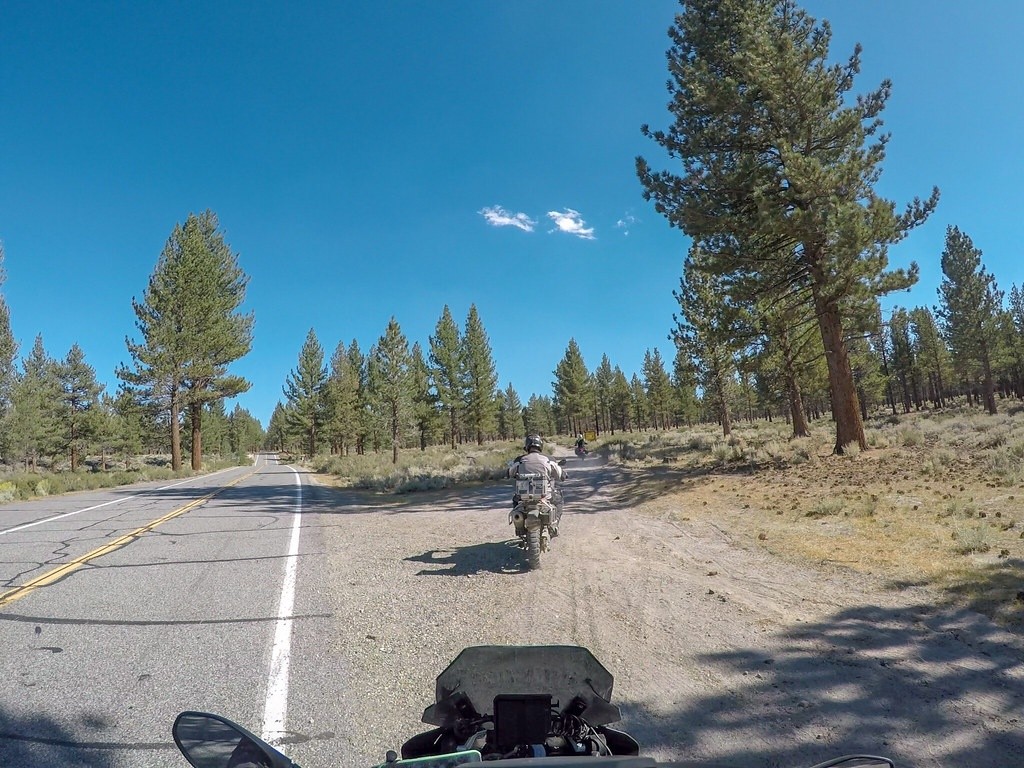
[565,472,568,479]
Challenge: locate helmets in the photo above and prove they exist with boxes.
[526,433,543,452]
[579,434,582,438]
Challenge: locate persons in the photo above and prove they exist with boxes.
[574,434,588,457]
[507,433,566,535]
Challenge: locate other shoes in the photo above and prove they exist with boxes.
[550,517,560,535]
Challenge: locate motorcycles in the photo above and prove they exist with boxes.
[504,455,569,570]
[574,442,589,462]
